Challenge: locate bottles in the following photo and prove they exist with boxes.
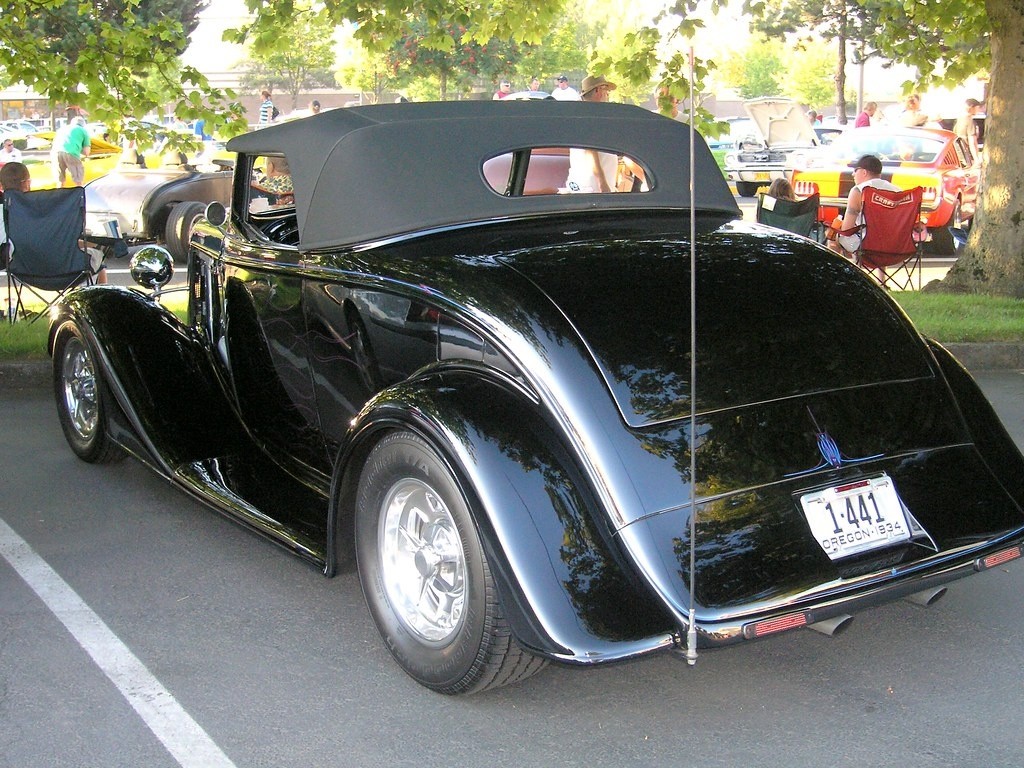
[827,215,844,239]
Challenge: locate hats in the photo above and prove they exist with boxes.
[313,100,321,107]
[846,155,883,174]
[579,73,617,99]
[557,76,568,82]
[70,116,85,126]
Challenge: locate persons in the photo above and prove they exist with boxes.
[169,113,238,142]
[103,133,110,142]
[56,117,92,188]
[899,95,944,127]
[854,102,876,127]
[827,154,901,291]
[309,101,321,114]
[953,98,986,163]
[253,157,294,193]
[767,178,852,260]
[142,132,173,162]
[622,80,696,192]
[806,110,821,125]
[567,74,618,193]
[120,117,138,155]
[256,91,272,129]
[551,76,582,101]
[493,79,512,100]
[0,162,107,287]
[0,139,22,164]
[525,79,539,91]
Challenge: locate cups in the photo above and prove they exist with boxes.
[102,218,122,238]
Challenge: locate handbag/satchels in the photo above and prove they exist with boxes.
[272,107,280,120]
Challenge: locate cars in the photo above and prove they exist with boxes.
[47,98,1023,698]
[702,96,986,249]
[0,116,236,264]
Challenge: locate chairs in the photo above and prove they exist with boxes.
[822,186,923,291]
[910,152,935,162]
[756,192,827,247]
[3,186,128,326]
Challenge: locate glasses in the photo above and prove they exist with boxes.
[6,143,14,147]
[854,168,862,172]
[504,84,510,88]
